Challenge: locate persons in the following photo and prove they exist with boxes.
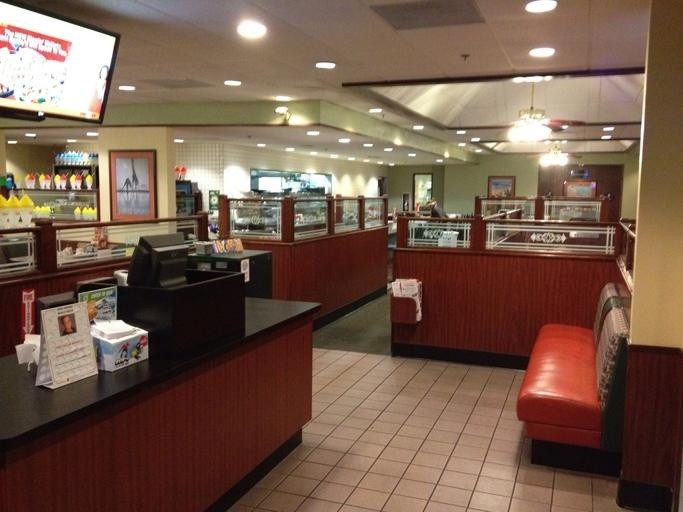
[117,341,129,358]
[426,200,449,219]
[87,299,115,323]
[131,342,145,360]
[59,314,77,335]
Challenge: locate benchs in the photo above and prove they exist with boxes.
[515,281,625,474]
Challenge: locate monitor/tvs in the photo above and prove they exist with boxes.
[127,232,189,289]
[0,0,121,124]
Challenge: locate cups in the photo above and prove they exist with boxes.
[0,173,97,226]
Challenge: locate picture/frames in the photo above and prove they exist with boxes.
[108,149,157,221]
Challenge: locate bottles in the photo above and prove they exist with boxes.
[55,150,98,166]
